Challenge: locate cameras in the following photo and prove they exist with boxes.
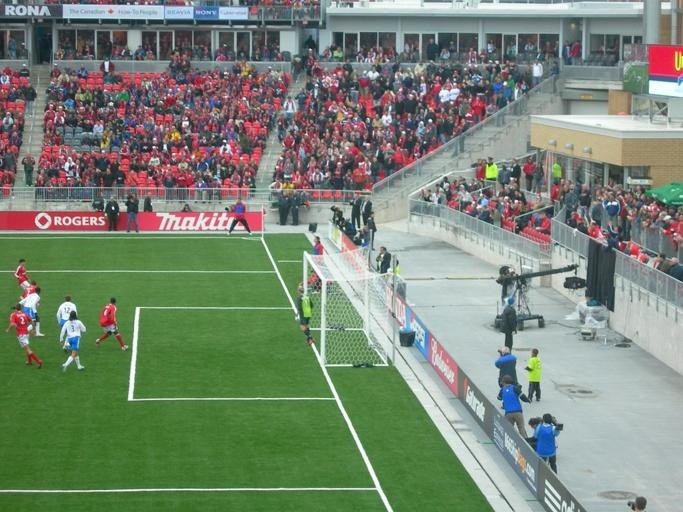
[498,349,502,355]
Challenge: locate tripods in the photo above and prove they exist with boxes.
[507,284,531,317]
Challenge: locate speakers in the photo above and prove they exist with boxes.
[309,222,317,232]
[399,330,416,347]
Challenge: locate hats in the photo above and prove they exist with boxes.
[504,296,514,305]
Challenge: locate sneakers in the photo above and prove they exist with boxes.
[25,362,32,366]
[308,337,315,345]
[121,344,128,351]
[62,364,66,373]
[77,366,85,370]
[36,333,45,336]
[37,361,42,369]
[95,339,103,348]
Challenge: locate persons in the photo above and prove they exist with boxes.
[19,280,36,299]
[294,286,314,345]
[532,413,559,475]
[499,298,517,353]
[633,496,647,512]
[1,1,582,236]
[581,176,682,282]
[312,236,323,281]
[94,297,128,352]
[13,259,30,289]
[521,348,542,402]
[494,347,517,387]
[58,311,86,372]
[495,374,530,439]
[374,245,391,275]
[391,260,401,275]
[527,417,558,448]
[19,288,44,337]
[55,296,78,352]
[4,304,42,369]
